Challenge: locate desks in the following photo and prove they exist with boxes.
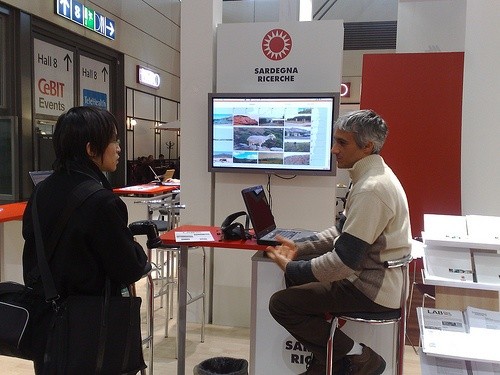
[111,181,180,222]
[0,200,29,281]
[156,224,283,375]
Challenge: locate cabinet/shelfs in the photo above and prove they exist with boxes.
[418,214,500,375]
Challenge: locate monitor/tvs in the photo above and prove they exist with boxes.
[207,92,337,176]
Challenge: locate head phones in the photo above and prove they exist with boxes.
[221,211,252,241]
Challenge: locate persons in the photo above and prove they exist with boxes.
[127,153,182,186]
[22,106,149,375]
[266,111,412,375]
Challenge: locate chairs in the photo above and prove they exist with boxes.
[325,254,413,375]
[127,218,207,351]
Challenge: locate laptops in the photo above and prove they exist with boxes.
[241,185,320,244]
[151,169,175,183]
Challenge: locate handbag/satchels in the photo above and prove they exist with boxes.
[46,296,145,375]
[0,281,52,360]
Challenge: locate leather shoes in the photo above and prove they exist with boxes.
[342,343,386,374]
[297,356,349,375]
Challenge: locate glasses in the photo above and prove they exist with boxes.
[111,139,121,145]
